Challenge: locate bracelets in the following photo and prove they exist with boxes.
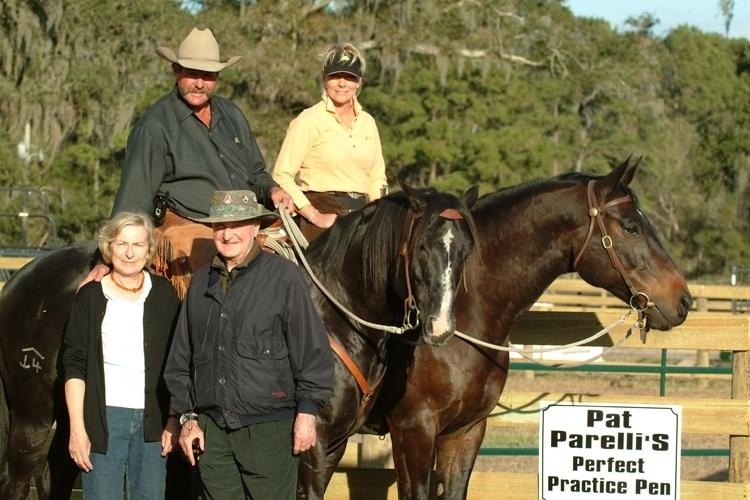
[308,208,319,222]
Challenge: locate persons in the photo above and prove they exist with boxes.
[56,210,181,500]
[76,26,295,292]
[271,40,389,245]
[162,189,337,500]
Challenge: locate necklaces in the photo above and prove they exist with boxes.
[110,268,146,293]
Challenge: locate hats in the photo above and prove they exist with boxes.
[155,28,241,72]
[323,49,364,79]
[189,190,281,229]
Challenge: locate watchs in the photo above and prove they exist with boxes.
[179,413,199,425]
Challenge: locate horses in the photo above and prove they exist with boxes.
[361,154,693,500]
[0,179,477,499]
